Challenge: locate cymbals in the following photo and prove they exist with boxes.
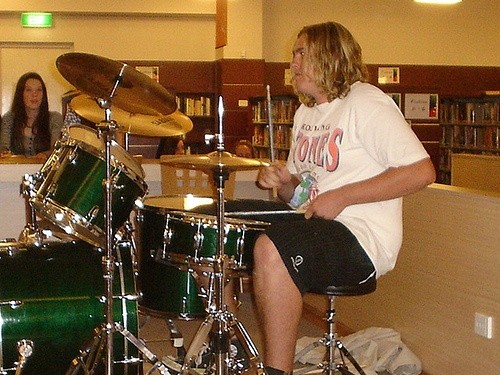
[68,124,146,178]
[162,153,269,171]
[54,51,177,116]
[69,94,194,135]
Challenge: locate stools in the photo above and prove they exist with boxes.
[293,277,377,375]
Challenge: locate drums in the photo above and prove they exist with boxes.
[139,256,206,320]
[0,237,141,375]
[139,193,289,273]
[28,139,150,250]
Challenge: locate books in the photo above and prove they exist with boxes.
[438,101,500,185]
[251,97,298,161]
[171,95,213,118]
[198,21,436,375]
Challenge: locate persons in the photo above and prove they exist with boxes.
[232,139,255,159]
[0,72,64,163]
[156,137,187,160]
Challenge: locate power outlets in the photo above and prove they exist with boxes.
[475,311,492,340]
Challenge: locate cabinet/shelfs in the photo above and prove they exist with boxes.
[175,91,500,186]
